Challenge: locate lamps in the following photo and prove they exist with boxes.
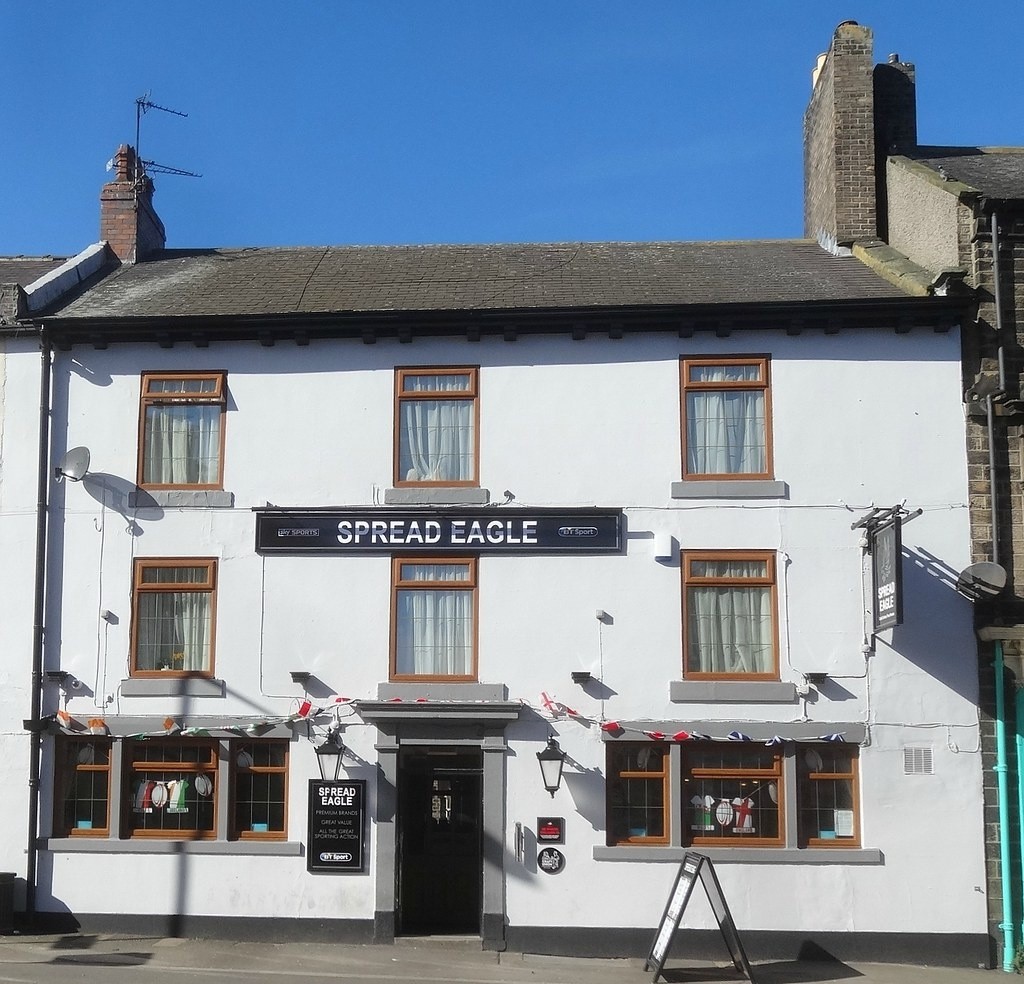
[289,671,310,683]
[803,672,828,685]
[537,732,566,797]
[313,727,345,782]
[570,672,590,683]
